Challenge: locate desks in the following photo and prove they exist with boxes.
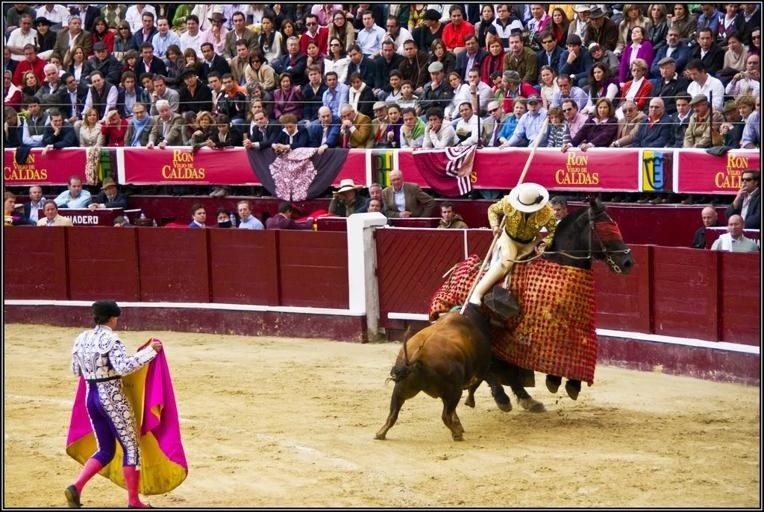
[705,224,760,253]
[313,213,442,232]
[34,204,142,228]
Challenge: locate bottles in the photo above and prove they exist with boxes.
[341,133,349,148]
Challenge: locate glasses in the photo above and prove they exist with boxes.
[741,177,755,183]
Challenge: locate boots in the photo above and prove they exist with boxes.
[468,266,503,306]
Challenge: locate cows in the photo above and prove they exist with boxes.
[373,300,496,443]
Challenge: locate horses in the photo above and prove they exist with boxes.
[427,194,635,414]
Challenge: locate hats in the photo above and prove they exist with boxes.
[90,6,719,115]
[336,177,364,194]
[99,177,118,189]
[507,181,552,214]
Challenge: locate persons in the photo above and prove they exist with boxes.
[469,182,556,306]
[73,299,162,508]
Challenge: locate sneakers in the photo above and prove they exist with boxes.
[63,484,83,507]
